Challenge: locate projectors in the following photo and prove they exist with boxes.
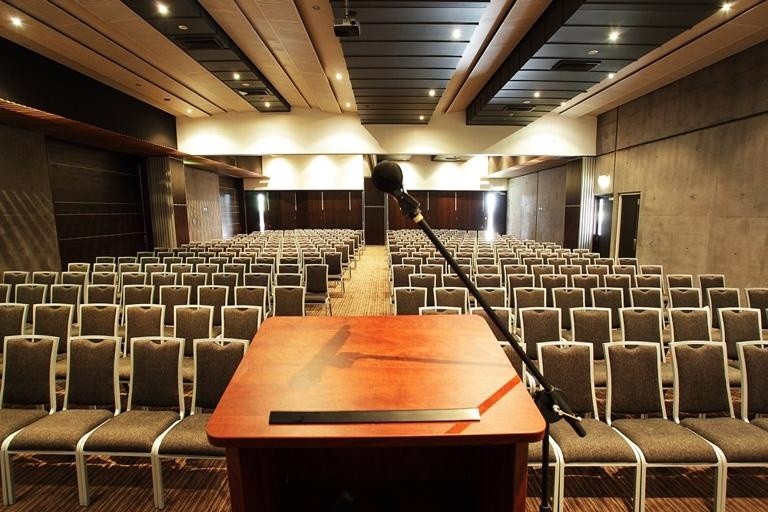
[332,18,362,39]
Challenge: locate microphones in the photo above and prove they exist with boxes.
[371,158,425,224]
